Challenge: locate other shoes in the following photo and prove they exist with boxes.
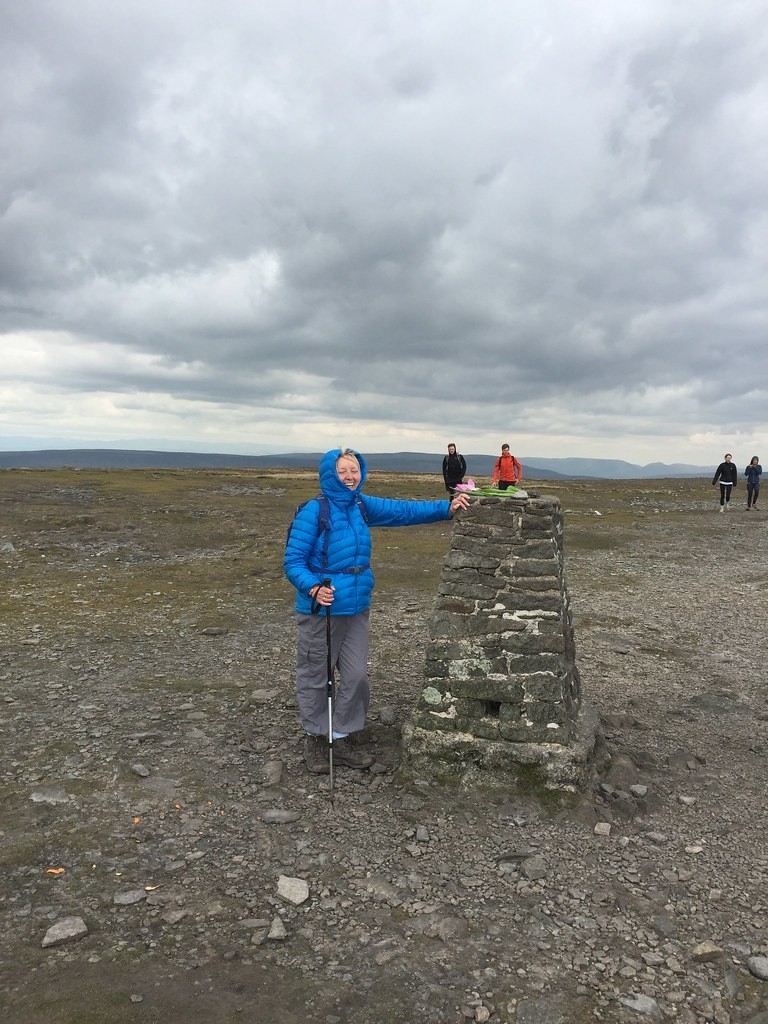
[746,507,751,511]
[752,503,759,511]
[726,504,730,511]
[720,506,724,512]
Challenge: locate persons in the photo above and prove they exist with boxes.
[712,454,737,513]
[490,443,522,490]
[285,450,471,775]
[442,443,467,502]
[745,456,762,512]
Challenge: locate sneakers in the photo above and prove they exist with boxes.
[326,735,375,768]
[304,737,330,775]
